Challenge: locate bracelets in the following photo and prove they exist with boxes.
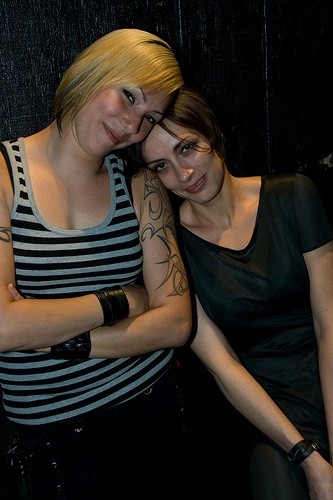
[51,331,93,359]
[93,286,129,327]
[285,439,319,465]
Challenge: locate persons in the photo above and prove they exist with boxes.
[1,28,195,500]
[129,88,332,500]
